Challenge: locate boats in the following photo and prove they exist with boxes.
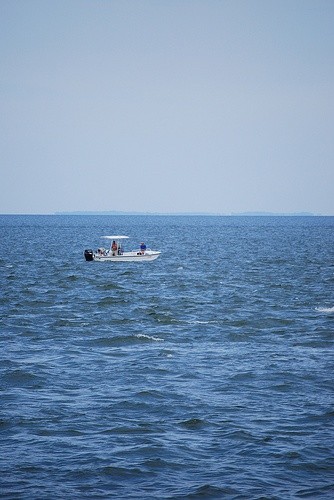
[83,235,163,262]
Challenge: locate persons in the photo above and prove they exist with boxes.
[111,241,117,256]
[140,242,147,255]
